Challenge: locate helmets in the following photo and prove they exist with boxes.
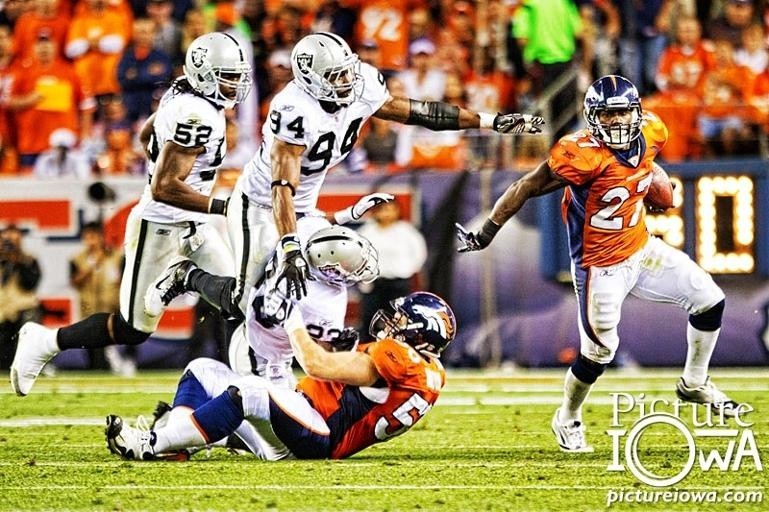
[304,226,381,291]
[290,32,365,107]
[582,75,642,150]
[368,291,457,358]
[183,32,253,110]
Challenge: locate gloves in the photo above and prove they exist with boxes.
[264,286,304,333]
[275,234,316,301]
[454,218,500,254]
[330,326,360,352]
[477,110,544,135]
[333,193,397,226]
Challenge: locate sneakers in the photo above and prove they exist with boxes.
[10,320,53,398]
[676,375,743,417]
[550,409,594,453]
[105,399,195,463]
[144,254,198,317]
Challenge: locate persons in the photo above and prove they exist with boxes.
[0,1,594,461]
[576,2,766,164]
[448,75,744,455]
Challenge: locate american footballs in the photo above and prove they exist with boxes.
[644,161,673,211]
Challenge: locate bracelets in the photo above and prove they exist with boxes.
[478,218,501,242]
